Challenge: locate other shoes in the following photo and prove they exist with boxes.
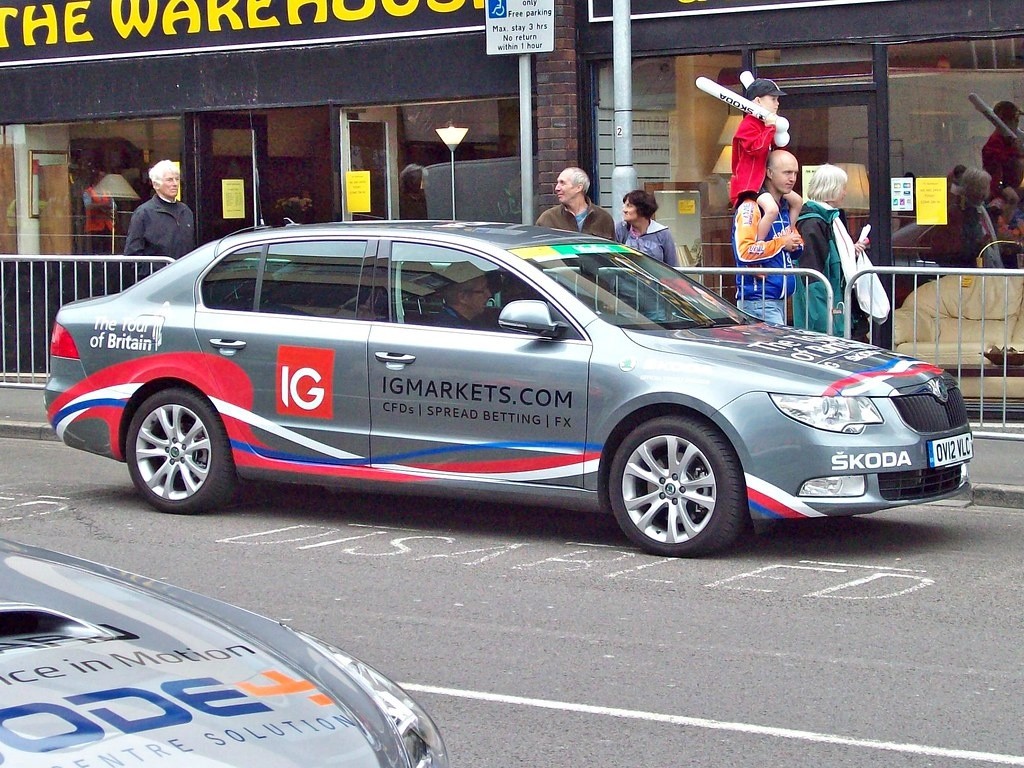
[746,262,767,279]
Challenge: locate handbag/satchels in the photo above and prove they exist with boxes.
[854,250,889,325]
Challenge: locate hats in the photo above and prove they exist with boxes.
[993,101,1024,117]
[744,77,788,101]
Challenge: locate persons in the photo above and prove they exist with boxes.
[897,101,1024,269]
[535,167,615,242]
[397,164,431,221]
[731,79,803,280]
[82,170,117,255]
[615,191,679,320]
[427,260,502,330]
[792,163,866,337]
[124,160,195,276]
[732,150,804,326]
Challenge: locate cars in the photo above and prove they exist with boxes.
[43,217,975,557]
[0,538,451,768]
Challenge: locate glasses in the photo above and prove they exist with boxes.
[460,286,489,294]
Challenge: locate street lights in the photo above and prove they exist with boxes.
[435,127,470,221]
